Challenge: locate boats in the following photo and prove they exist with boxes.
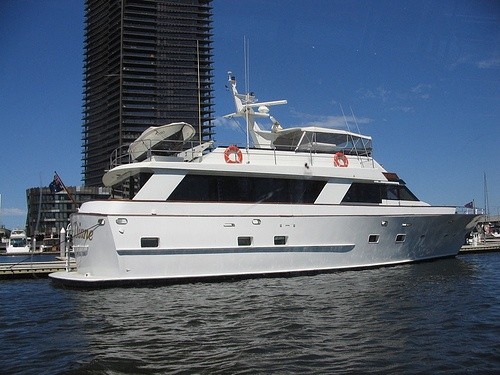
[5,228,31,255]
[459,221,500,252]
[45,35,484,289]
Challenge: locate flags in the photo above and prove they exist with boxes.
[48,174,64,192]
[464,201,473,209]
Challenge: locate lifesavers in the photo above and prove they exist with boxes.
[334,152,348,167]
[224,145,242,164]
